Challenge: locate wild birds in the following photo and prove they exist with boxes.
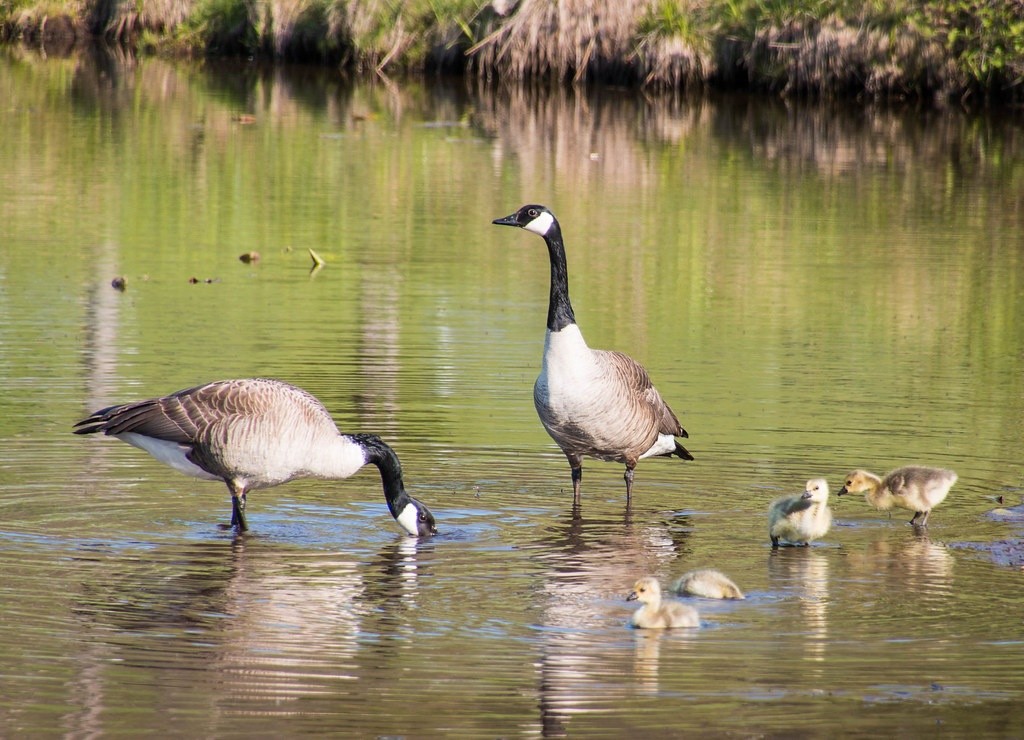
[626,576,700,629]
[669,568,746,600]
[71,378,439,539]
[492,204,695,516]
[837,464,959,526]
[767,477,832,550]
